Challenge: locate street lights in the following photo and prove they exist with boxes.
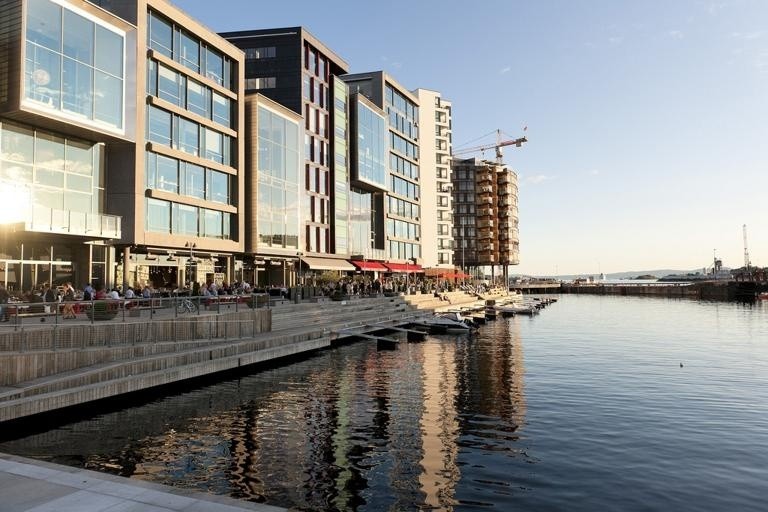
[185,241,197,303]
[436,264,439,285]
[405,261,409,289]
[296,252,304,286]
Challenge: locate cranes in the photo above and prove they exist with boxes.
[451,129,528,164]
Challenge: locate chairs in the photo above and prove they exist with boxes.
[10,311,62,327]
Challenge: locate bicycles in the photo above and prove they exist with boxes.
[175,297,197,314]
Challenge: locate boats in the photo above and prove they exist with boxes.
[410,316,480,335]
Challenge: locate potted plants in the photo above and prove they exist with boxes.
[85,302,118,320]
[245,294,268,308]
